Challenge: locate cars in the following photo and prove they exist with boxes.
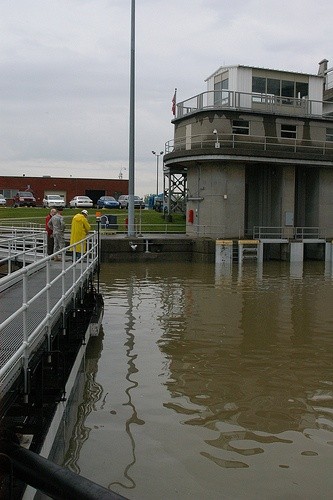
[0,194,8,206]
[97,196,120,209]
[69,195,93,209]
[42,195,65,210]
[15,191,37,208]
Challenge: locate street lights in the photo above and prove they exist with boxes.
[151,150,163,196]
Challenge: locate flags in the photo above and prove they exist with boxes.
[171,95,176,115]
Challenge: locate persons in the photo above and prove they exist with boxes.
[47,207,69,262]
[69,210,91,262]
[45,209,56,260]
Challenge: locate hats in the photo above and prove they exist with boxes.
[81,210,88,216]
[57,208,62,211]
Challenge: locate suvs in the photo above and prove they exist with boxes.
[117,195,146,209]
